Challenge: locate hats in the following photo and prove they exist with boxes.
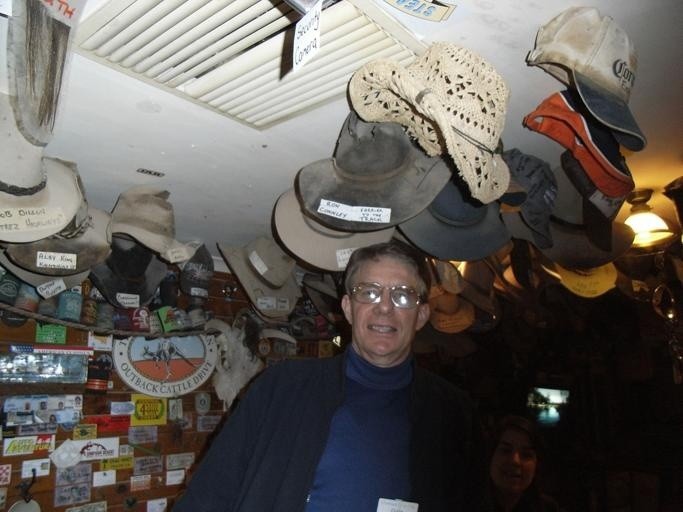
[0,157,213,309]
[216,7,648,332]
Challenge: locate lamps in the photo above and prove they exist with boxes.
[622,188,677,251]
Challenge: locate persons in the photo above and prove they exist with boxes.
[169,237,482,512]
[483,407,566,511]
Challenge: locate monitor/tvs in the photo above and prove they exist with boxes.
[525,385,573,418]
